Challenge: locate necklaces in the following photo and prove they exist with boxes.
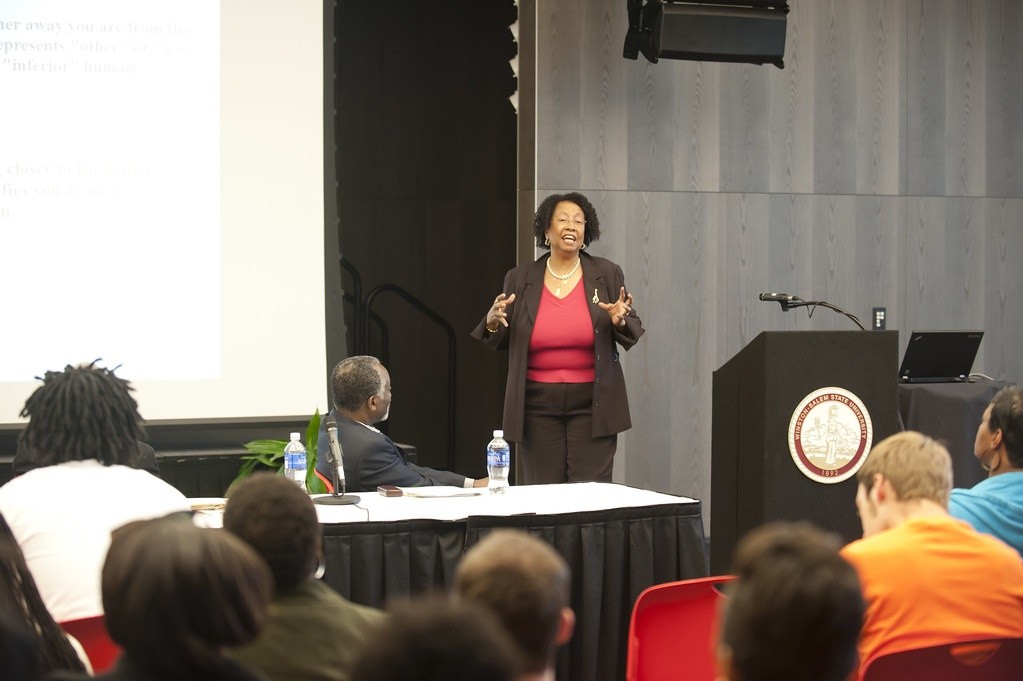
[547,256,580,286]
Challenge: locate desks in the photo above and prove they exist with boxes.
[184,483,708,681]
[896,380,1015,490]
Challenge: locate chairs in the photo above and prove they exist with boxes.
[59,614,124,676]
[625,574,736,681]
[859,637,1023,681]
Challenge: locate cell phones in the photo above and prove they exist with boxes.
[377,486,403,497]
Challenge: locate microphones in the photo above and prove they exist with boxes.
[323,416,345,487]
[759,292,800,301]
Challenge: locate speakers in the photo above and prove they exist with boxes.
[639,2,787,65]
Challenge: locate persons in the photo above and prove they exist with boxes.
[470,190,646,487]
[317,356,489,494]
[93,471,574,681]
[716,383,1023,681]
[0,357,209,626]
[0,509,95,681]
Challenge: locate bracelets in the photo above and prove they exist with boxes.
[486,325,500,332]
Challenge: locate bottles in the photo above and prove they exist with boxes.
[284,432,307,493]
[487,430,510,496]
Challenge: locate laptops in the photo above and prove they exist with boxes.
[899,330,984,384]
